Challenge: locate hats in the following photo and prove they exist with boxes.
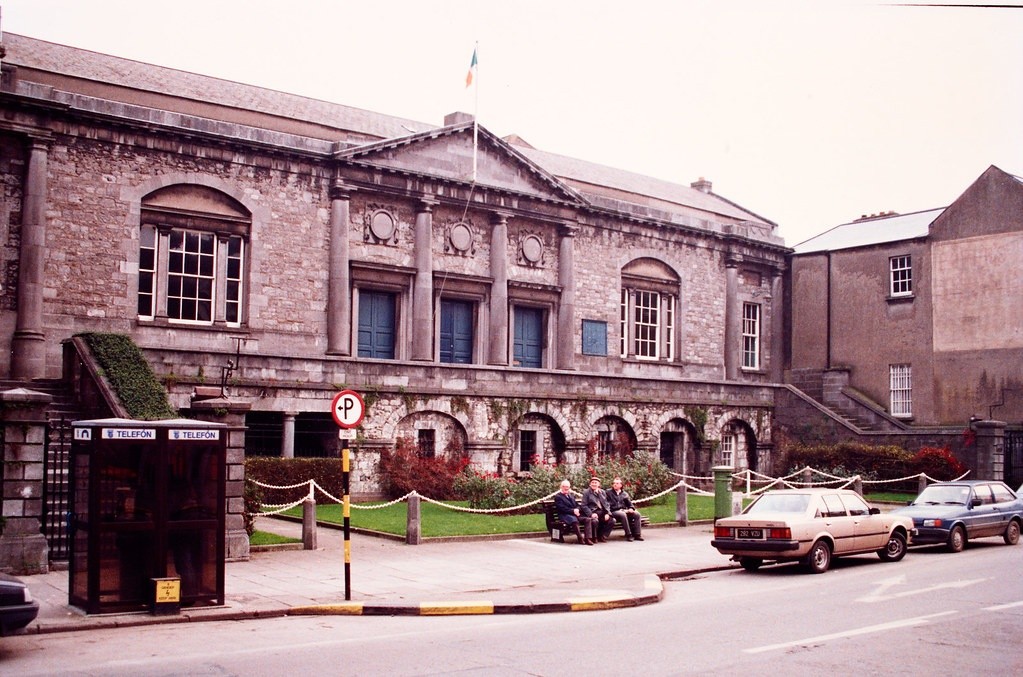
[590,477,600,482]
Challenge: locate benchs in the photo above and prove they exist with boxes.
[537,499,650,543]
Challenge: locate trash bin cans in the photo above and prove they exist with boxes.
[711,465,735,524]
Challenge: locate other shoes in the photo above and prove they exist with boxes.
[633,534,644,541]
[584,538,593,545]
[598,535,606,542]
[593,537,598,543]
[627,534,634,542]
[579,538,585,544]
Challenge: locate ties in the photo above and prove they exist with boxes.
[595,492,598,496]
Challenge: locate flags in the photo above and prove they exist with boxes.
[466,44,477,88]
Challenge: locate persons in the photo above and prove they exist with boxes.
[554,477,644,545]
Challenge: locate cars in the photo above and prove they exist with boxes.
[711,489,915,573]
[887,480,1023,552]
[0,574,40,637]
